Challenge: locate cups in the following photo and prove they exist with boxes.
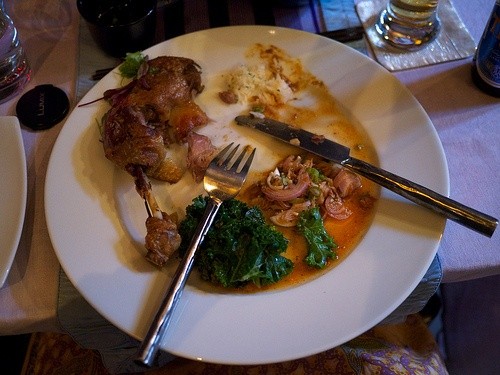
[0,2,30,102]
[377,0,440,49]
[76,0,160,57]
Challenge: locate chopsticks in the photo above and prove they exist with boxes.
[94,25,364,80]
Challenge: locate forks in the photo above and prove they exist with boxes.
[131,142,257,368]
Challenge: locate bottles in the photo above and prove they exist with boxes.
[471,0,500,98]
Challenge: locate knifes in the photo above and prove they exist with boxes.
[235,115,499,238]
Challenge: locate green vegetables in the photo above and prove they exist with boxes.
[116,51,146,84]
[179,194,294,290]
[297,167,338,269]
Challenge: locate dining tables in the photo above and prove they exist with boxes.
[0,0,500,336]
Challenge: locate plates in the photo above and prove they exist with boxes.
[0,114,25,289]
[44,25,453,364]
